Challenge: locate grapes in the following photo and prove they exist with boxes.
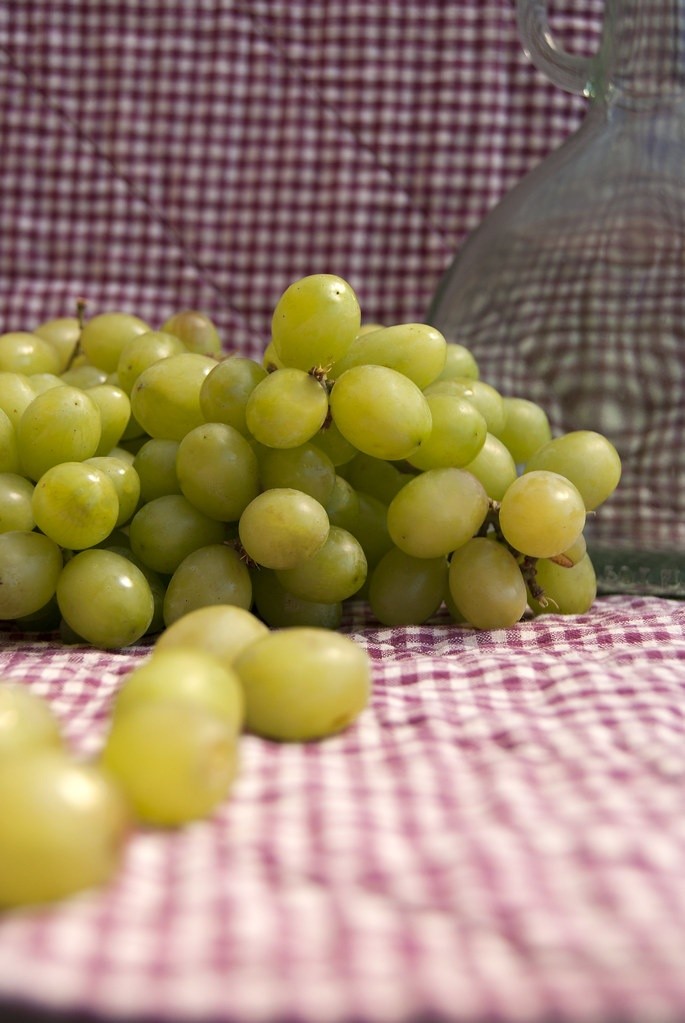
[0,273,622,912]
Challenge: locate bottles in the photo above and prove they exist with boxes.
[428,0,685,595]
